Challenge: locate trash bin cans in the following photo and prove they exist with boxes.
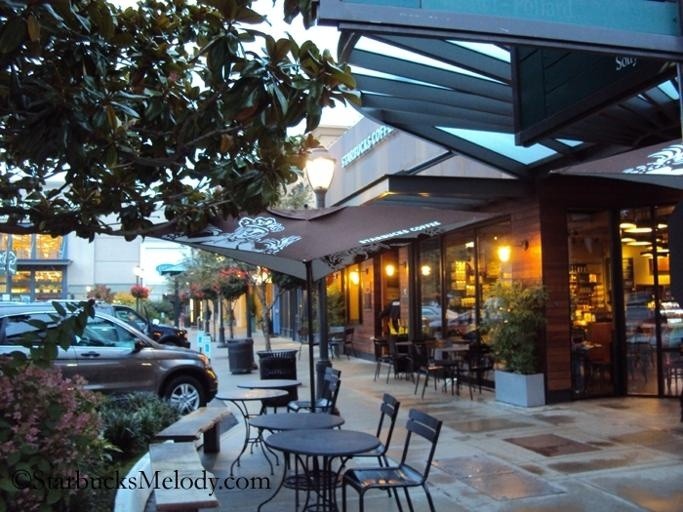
[257,349,299,407]
[228,339,253,374]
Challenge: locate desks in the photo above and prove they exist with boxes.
[257,429,380,512]
[249,413,347,489]
[215,389,289,479]
[237,379,301,454]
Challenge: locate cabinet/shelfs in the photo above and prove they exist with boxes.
[570,261,603,304]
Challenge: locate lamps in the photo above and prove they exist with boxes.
[385,261,407,276]
[349,268,369,285]
[496,240,528,262]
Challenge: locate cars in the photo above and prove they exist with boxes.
[411,298,508,338]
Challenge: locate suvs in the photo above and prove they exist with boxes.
[0,299,218,416]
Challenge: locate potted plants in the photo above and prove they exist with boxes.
[478,269,548,408]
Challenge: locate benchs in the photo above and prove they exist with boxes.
[156,407,230,454]
[149,442,219,512]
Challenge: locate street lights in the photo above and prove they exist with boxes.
[304,141,337,408]
[133,264,141,314]
[140,268,146,316]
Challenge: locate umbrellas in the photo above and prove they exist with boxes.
[137,199,511,415]
[548,141,683,188]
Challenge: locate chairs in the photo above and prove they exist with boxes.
[308,377,340,414]
[296,328,494,400]
[342,408,443,512]
[329,393,403,512]
[287,367,342,414]
